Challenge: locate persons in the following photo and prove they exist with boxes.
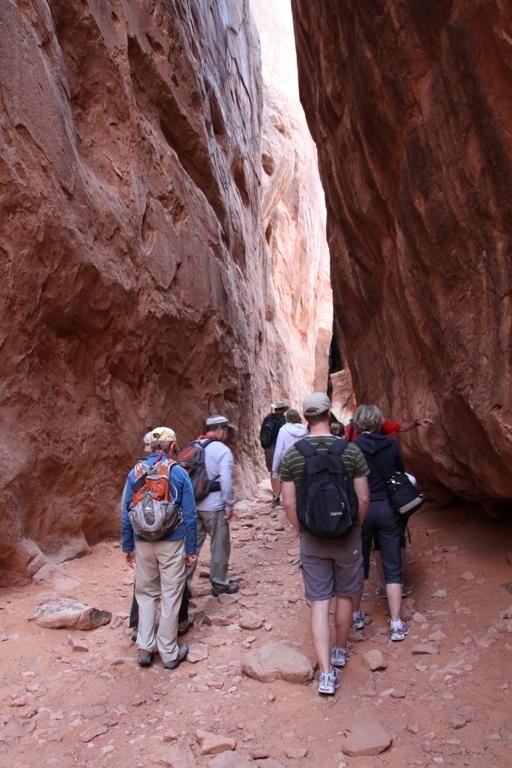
[180,413,239,597]
[259,391,437,695]
[122,426,198,669]
[127,564,195,643]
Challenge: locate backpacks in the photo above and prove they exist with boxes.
[128,460,181,541]
[295,439,358,537]
[261,415,282,448]
[177,439,220,501]
[385,472,423,515]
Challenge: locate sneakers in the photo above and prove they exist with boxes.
[376,584,387,598]
[273,495,281,505]
[401,582,413,597]
[213,582,238,596]
[179,614,193,634]
[132,628,137,640]
[137,650,152,665]
[331,646,350,666]
[319,667,342,693]
[387,618,409,640]
[351,609,364,630]
[163,643,188,668]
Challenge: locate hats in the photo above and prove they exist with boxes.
[303,393,329,416]
[143,427,181,451]
[271,402,288,409]
[206,415,236,429]
